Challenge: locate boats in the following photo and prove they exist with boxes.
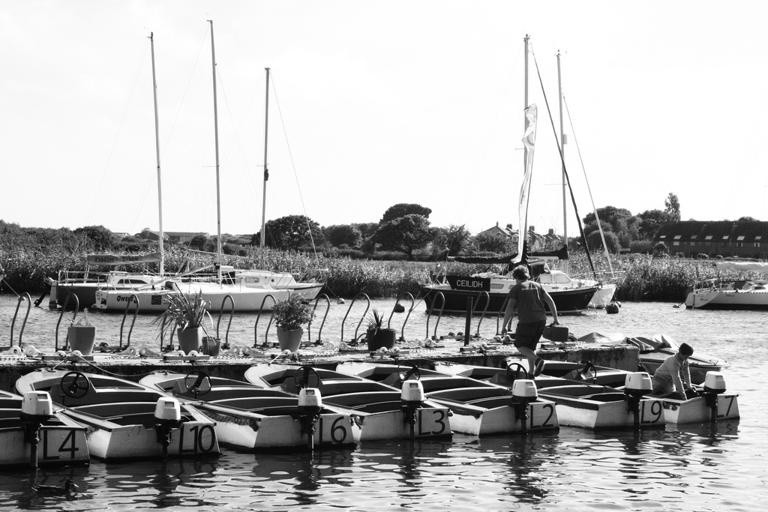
[0,388,92,465]
[14,360,223,454]
[433,360,669,432]
[591,332,723,387]
[685,280,768,310]
[505,356,742,426]
[136,368,357,453]
[243,361,456,443]
[335,360,559,436]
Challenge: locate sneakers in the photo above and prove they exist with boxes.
[527,360,545,379]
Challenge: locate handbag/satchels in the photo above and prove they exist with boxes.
[543,321,568,343]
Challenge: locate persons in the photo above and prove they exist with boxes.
[644,342,697,401]
[501,265,560,379]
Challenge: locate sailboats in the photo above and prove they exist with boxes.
[415,34,625,316]
[49,20,324,314]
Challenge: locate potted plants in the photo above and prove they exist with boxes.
[270,289,317,350]
[150,288,214,354]
[352,308,395,356]
[68,307,95,354]
